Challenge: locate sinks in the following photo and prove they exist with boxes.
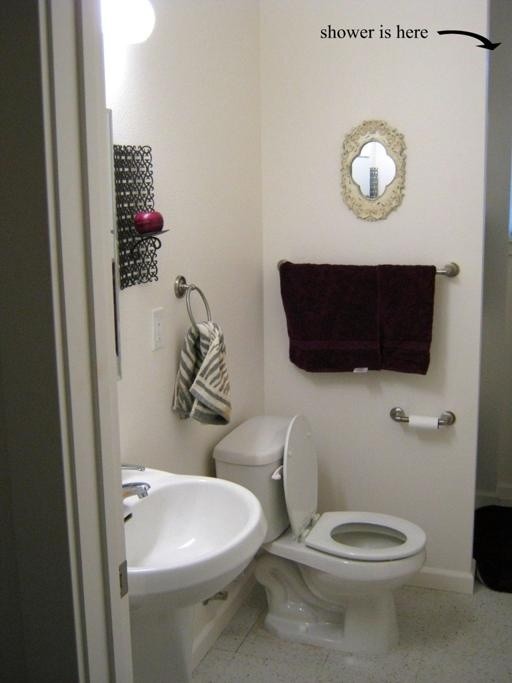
[122,466,268,598]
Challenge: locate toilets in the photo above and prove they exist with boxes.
[214,415,428,657]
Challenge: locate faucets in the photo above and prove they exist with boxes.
[121,480,151,500]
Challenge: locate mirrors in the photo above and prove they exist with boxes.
[339,116,409,223]
[105,107,125,384]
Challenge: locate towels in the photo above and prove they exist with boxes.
[280,259,384,373]
[376,263,438,375]
[169,318,235,422]
